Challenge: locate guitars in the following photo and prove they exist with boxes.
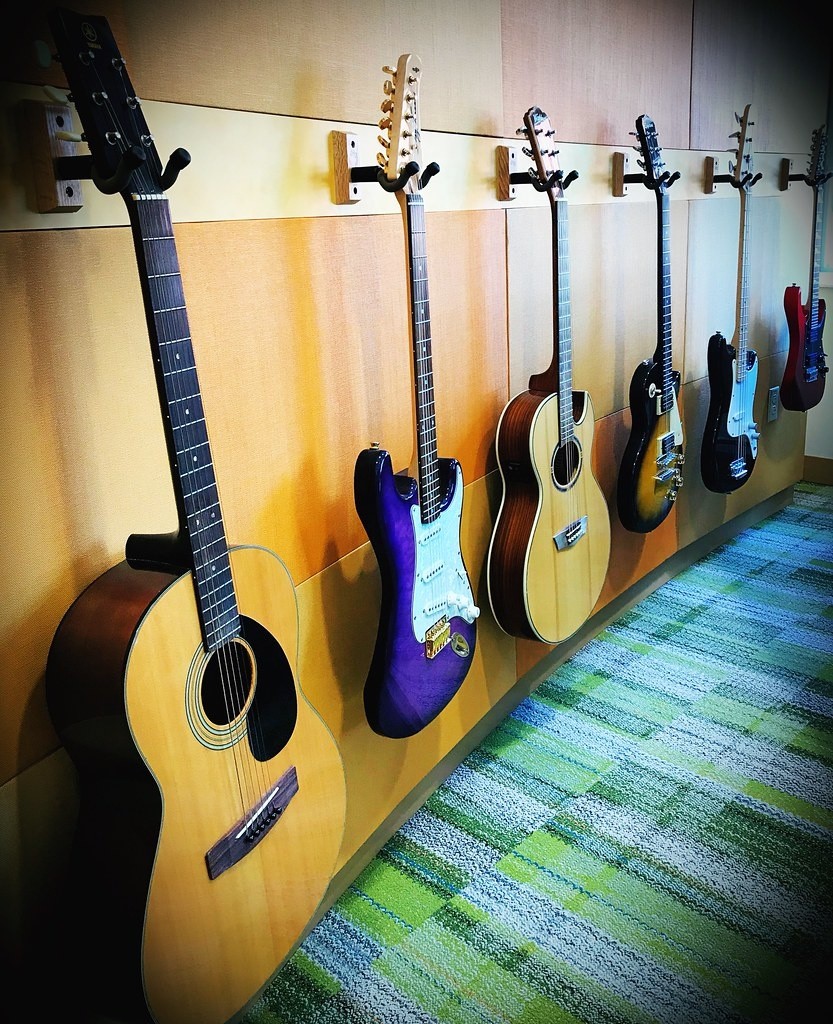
[353,55,481,739]
[779,123,826,411]
[486,104,613,645]
[45,36,346,1023]
[701,104,760,493]
[616,112,687,532]
